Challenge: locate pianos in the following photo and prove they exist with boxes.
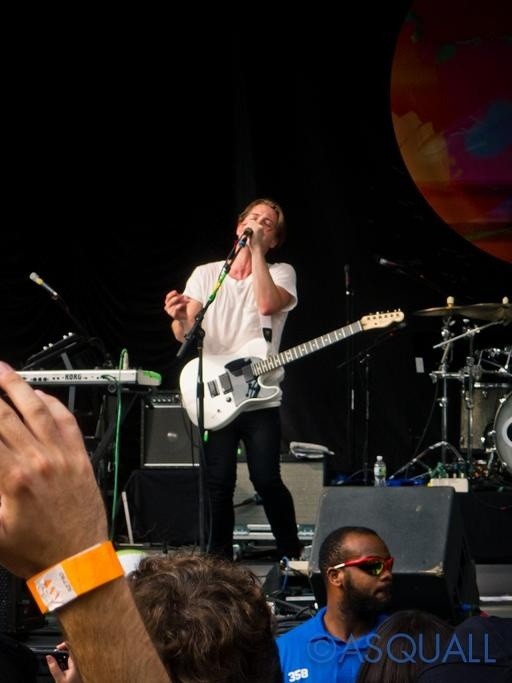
[13,369,161,387]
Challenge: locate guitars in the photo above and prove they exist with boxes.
[180,310,404,431]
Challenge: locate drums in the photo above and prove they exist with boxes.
[492,386,512,474]
[488,390,511,472]
[473,348,512,389]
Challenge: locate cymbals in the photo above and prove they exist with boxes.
[416,306,464,316]
[460,303,511,322]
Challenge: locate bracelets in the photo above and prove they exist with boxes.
[25,539,126,616]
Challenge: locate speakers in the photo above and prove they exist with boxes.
[310,486,467,605]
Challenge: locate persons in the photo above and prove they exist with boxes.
[356,607,447,683]
[0,362,170,683]
[126,546,285,682]
[275,527,395,682]
[162,199,302,561]
[0,633,81,683]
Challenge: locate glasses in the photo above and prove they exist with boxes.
[333,556,394,575]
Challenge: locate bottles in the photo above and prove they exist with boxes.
[374,456,387,486]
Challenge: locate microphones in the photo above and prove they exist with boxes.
[29,271,62,302]
[236,226,252,249]
